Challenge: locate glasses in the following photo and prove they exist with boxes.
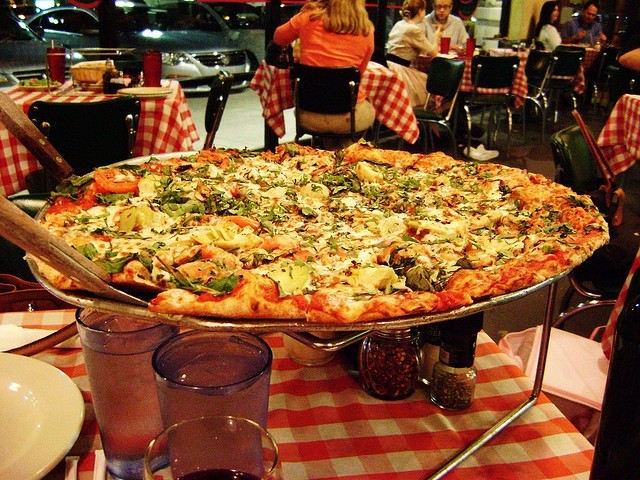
[434,4,451,10]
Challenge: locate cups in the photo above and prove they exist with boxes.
[145,413,278,478]
[47,47,67,85]
[143,51,162,88]
[152,332,273,480]
[74,307,182,480]
[441,36,452,54]
[466,37,476,58]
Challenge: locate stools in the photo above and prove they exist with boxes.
[524,319,613,433]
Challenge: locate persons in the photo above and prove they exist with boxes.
[417,0,486,137]
[273,1,375,86]
[560,2,607,46]
[384,0,443,111]
[534,1,562,53]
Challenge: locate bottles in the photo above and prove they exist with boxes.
[102,59,118,96]
[429,322,477,410]
[359,328,421,399]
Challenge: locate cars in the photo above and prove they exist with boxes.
[22,9,98,64]
[115,5,259,96]
[2,8,87,91]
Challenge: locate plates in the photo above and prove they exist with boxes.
[0,350,85,480]
[19,82,63,90]
[117,88,173,99]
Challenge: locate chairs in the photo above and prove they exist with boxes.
[550,242,632,330]
[372,57,467,160]
[461,55,521,156]
[24,93,140,196]
[193,69,234,151]
[586,47,619,117]
[550,126,614,205]
[547,45,586,121]
[285,64,380,153]
[493,50,554,149]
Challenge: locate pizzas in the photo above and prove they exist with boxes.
[26,143,612,324]
